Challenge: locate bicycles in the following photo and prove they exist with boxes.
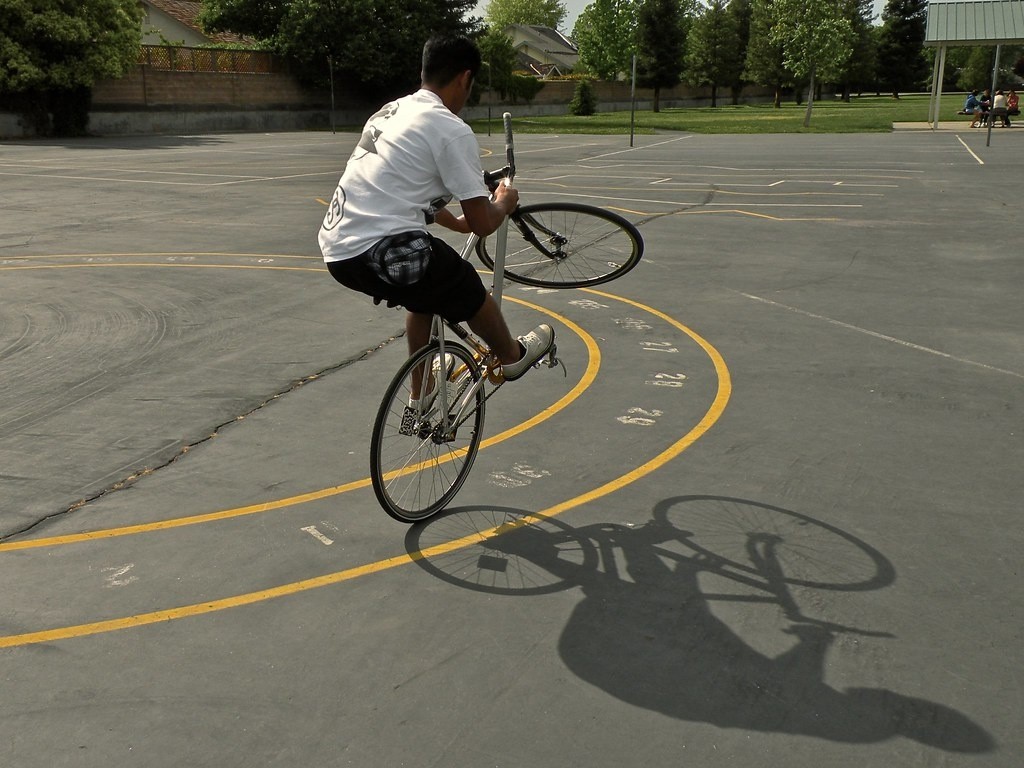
[368,111,646,524]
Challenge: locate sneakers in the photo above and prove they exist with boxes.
[409,352,456,415]
[500,323,555,382]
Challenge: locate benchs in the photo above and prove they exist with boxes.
[957,110,1021,128]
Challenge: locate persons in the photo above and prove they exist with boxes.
[965,89,1019,128]
[319,33,556,416]
